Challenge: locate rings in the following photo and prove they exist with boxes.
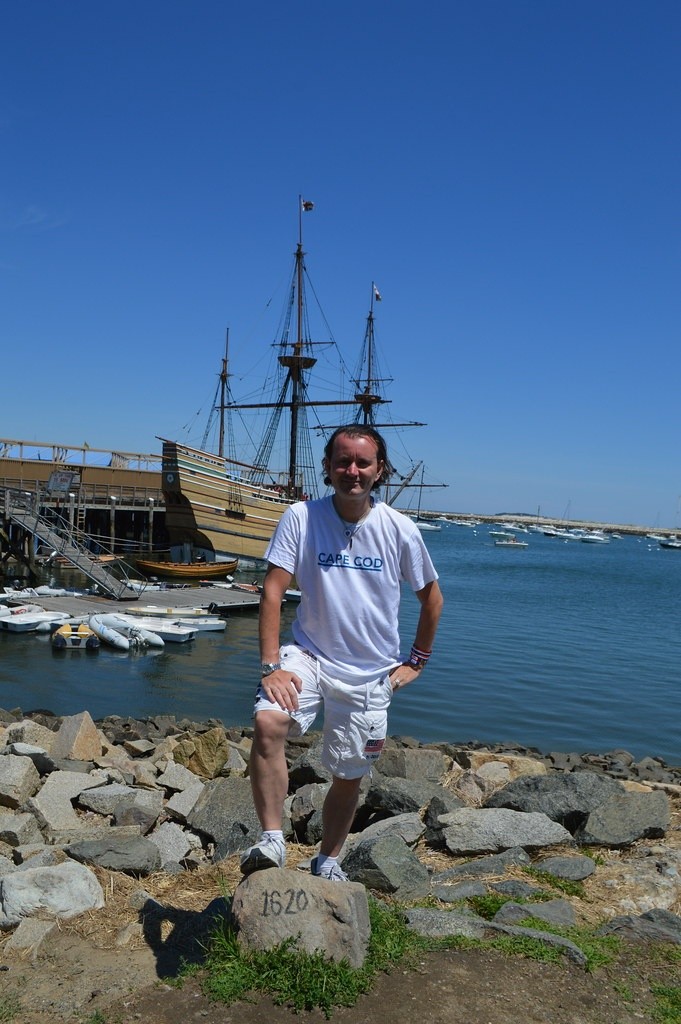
[395,680,399,686]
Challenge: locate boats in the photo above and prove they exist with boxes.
[496,537,527,549]
[54,553,124,568]
[611,532,625,541]
[490,520,610,544]
[412,521,440,532]
[0,580,308,648]
[648,532,665,540]
[659,537,681,551]
[407,515,483,529]
[138,556,240,581]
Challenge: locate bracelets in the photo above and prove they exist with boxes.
[409,644,432,669]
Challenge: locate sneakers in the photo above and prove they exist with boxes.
[240,833,286,873]
[311,857,348,882]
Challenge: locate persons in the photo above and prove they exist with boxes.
[239,424,444,883]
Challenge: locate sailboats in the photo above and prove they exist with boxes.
[159,238,355,569]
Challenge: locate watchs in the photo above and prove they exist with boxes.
[261,663,281,676]
